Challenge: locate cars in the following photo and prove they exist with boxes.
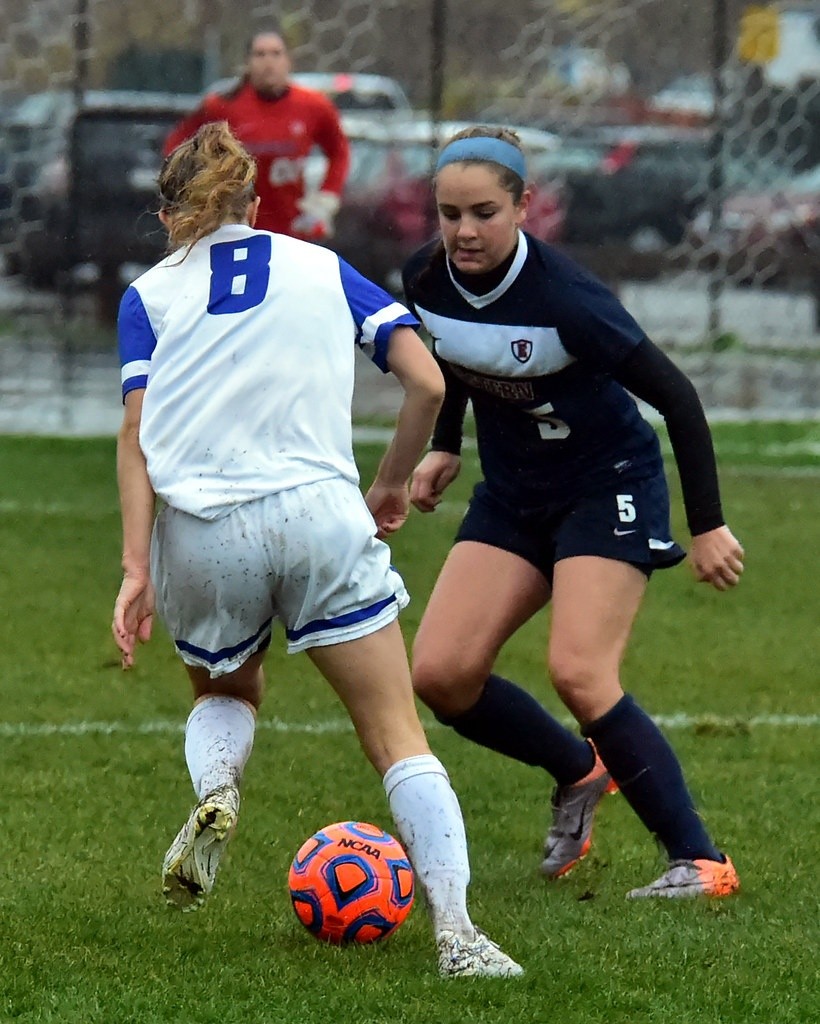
[213,70,820,295]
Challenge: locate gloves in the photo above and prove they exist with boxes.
[294,191,340,238]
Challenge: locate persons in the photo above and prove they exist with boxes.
[112,120,526,980]
[159,27,348,243]
[402,128,744,898]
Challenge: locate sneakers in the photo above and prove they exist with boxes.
[162,784,241,914]
[625,853,741,904]
[436,924,525,981]
[538,737,618,882]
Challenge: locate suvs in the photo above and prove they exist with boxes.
[0,89,206,299]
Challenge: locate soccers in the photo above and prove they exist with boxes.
[287,819,416,948]
